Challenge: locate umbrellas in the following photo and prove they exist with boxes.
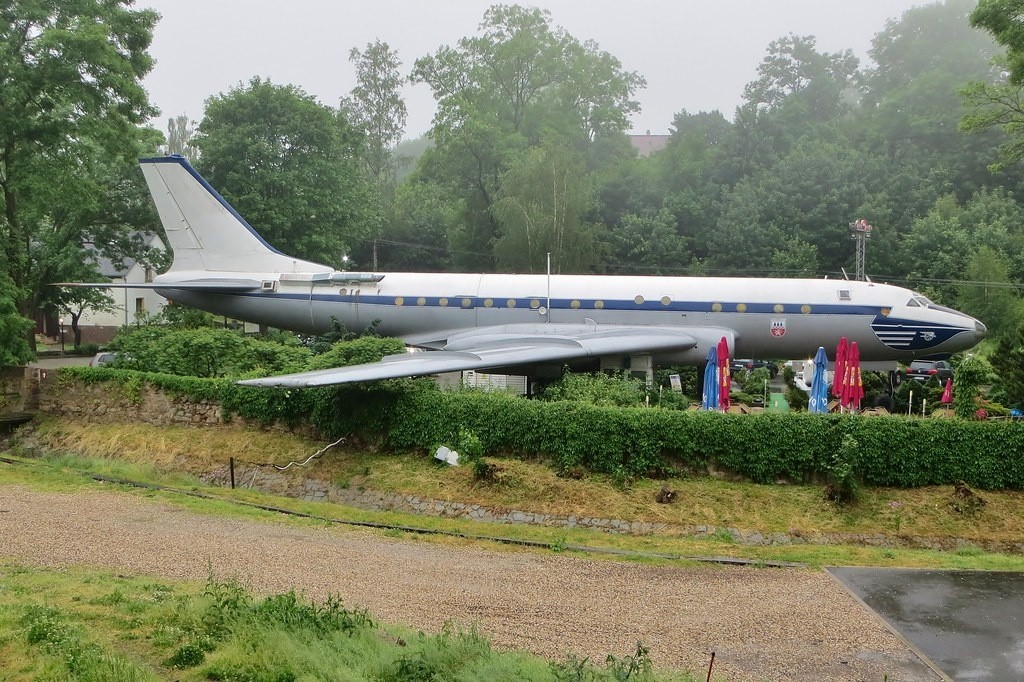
[942,377,953,410]
[808,346,828,413]
[842,342,864,414]
[716,336,730,414]
[702,346,719,411]
[831,336,850,412]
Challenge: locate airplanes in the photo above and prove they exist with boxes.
[45,154,988,388]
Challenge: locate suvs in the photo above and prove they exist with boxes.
[730,356,779,380]
[905,359,955,387]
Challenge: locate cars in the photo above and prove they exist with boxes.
[782,359,836,386]
